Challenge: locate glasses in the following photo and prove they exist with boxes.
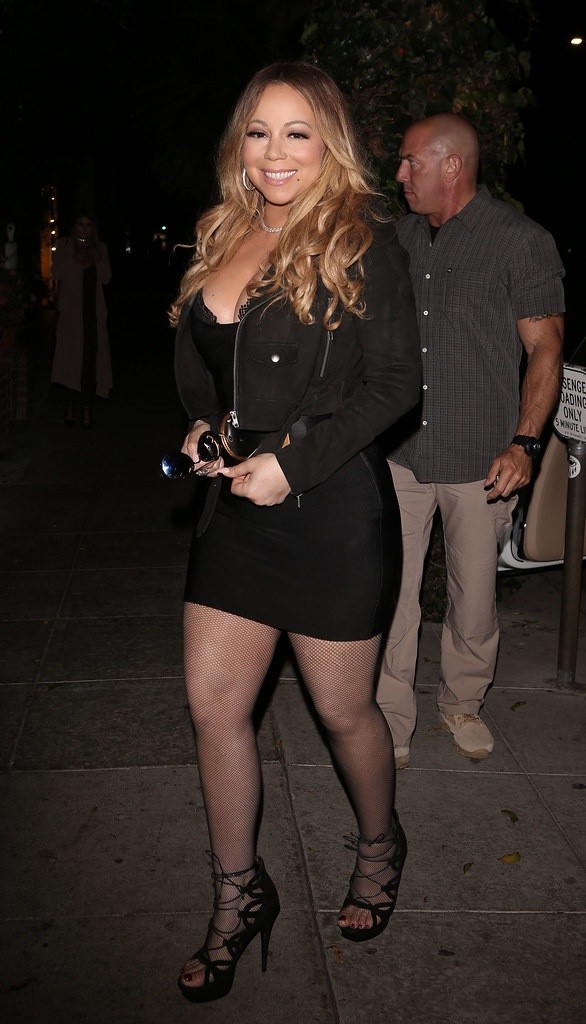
[160,431,224,482]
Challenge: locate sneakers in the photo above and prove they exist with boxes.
[438,710,496,759]
[393,747,409,769]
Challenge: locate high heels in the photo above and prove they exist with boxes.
[178,850,280,1003]
[337,809,408,941]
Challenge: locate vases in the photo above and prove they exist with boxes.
[0,328,16,351]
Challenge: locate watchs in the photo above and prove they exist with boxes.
[512,435,541,456]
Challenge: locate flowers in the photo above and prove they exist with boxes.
[0,255,48,337]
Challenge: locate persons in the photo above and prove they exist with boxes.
[52,213,112,429]
[170,60,406,1002]
[376,113,565,769]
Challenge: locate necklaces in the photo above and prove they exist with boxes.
[261,222,282,232]
